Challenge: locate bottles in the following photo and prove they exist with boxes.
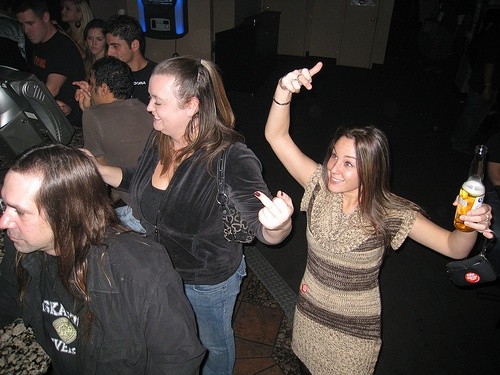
[453,145,488,231]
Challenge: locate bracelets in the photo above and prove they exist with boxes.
[272,95,292,105]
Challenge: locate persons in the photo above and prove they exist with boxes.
[486,111,500,188]
[78,54,296,375]
[59,0,95,59]
[1,139,209,375]
[83,18,109,83]
[102,14,159,106]
[13,0,85,130]
[264,61,495,375]
[72,55,154,236]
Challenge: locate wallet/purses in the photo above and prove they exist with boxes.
[446,231,497,288]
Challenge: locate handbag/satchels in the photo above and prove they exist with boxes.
[215,142,255,244]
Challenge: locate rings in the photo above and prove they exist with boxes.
[489,231,494,240]
[486,214,489,221]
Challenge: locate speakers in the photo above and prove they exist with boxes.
[138,0,189,40]
